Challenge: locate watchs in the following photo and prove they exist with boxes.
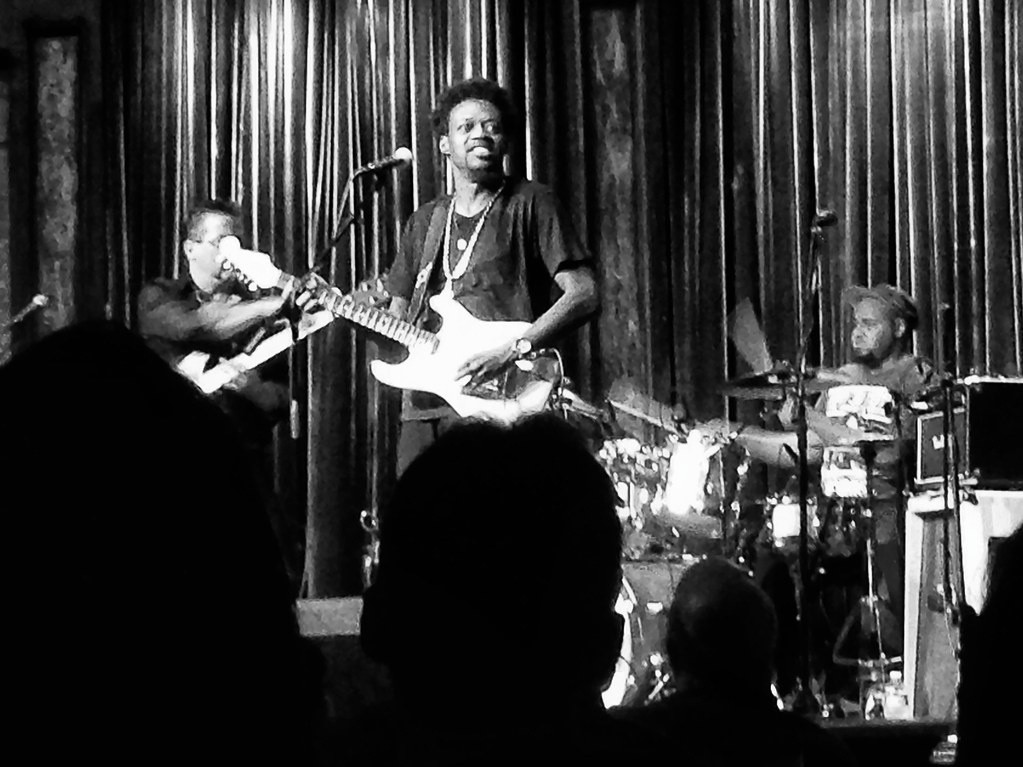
[511,336,532,359]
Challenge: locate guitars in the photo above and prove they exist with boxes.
[173,236,562,427]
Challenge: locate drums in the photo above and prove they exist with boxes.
[666,555,801,711]
[653,422,745,535]
[601,562,690,707]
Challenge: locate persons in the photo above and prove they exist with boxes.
[0,318,328,767]
[608,560,857,767]
[292,76,603,480]
[345,413,624,767]
[732,283,942,645]
[139,197,294,428]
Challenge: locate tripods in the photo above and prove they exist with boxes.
[824,447,908,718]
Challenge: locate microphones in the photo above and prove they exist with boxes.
[355,145,414,172]
[812,209,838,230]
[1,294,50,336]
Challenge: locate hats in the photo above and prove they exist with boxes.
[842,282,921,327]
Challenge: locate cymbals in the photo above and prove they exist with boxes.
[715,368,841,399]
[558,386,604,420]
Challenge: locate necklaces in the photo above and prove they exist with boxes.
[440,180,505,299]
